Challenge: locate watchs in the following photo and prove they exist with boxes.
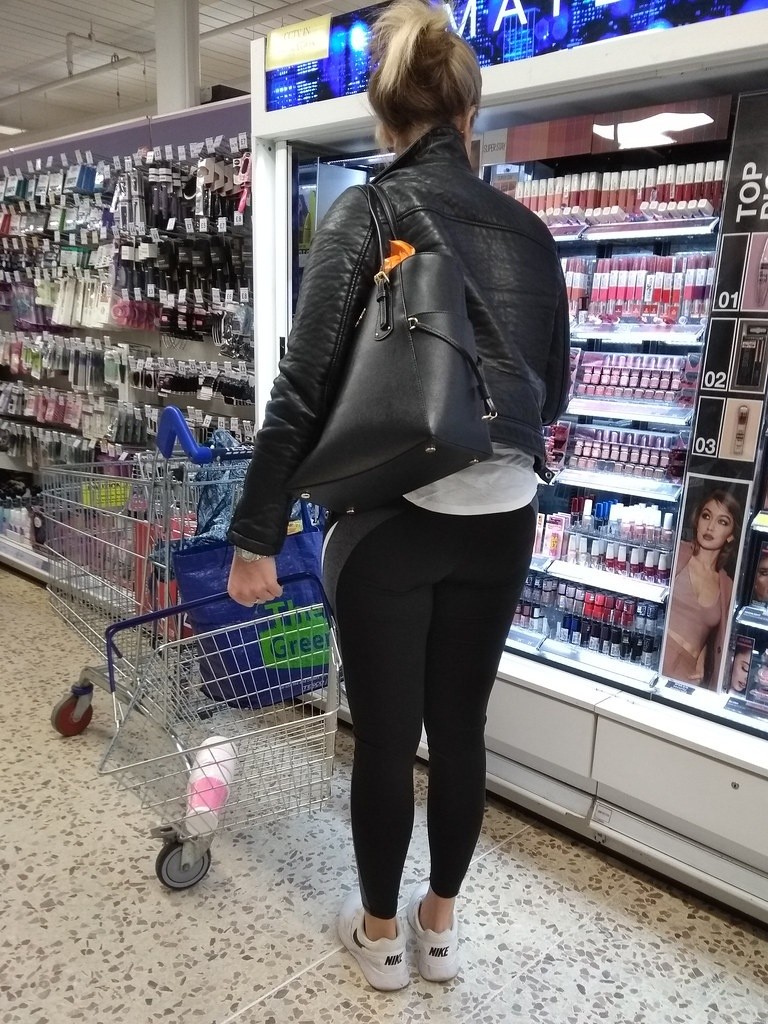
[233,545,267,562]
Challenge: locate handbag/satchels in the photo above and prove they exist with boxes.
[294,184,500,513]
[172,517,344,711]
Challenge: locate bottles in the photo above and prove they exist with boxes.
[0,480,48,545]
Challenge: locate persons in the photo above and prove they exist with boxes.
[663,489,742,689]
[730,647,751,692]
[227,0,572,991]
[753,552,768,603]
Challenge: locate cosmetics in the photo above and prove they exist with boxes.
[734,406,748,453]
[757,238,768,306]
[734,334,767,386]
[513,159,728,668]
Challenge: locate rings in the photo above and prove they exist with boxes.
[251,599,258,604]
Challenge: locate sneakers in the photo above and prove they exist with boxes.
[407,881,459,981]
[337,892,410,991]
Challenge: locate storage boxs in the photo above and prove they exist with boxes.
[266,0,768,113]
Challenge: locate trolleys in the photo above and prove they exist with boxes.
[30,405,349,892]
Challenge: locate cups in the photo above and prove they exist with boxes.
[185,736,238,837]
